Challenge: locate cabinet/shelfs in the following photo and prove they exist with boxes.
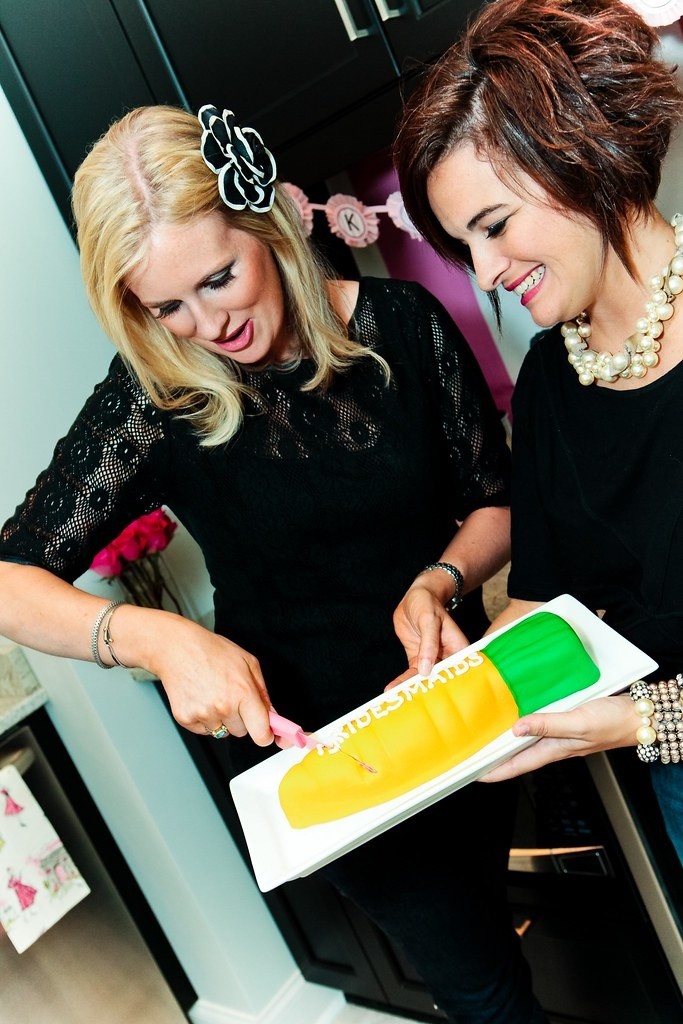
[152,679,683,1024]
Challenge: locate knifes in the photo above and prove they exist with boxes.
[267,712,378,776]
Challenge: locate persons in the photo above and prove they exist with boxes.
[394,0,682,865]
[1,105,683,1024]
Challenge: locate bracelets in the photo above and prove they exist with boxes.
[629,675,683,764]
[92,599,134,670]
[420,562,463,611]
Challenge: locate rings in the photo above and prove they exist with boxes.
[207,724,226,739]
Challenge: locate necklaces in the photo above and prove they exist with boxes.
[562,213,682,386]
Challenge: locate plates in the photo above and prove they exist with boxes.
[229,590,659,893]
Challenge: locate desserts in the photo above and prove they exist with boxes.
[275,611,601,830]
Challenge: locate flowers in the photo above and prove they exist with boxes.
[199,104,278,213]
[88,507,185,617]
